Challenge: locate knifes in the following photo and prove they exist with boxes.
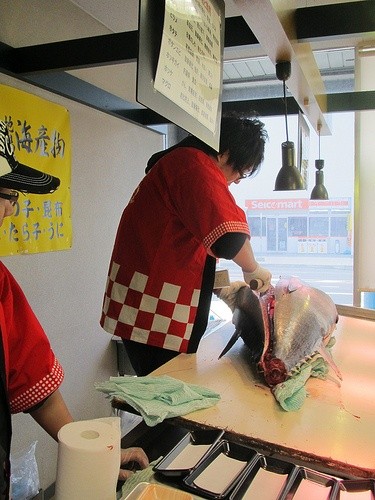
[249,278,267,297]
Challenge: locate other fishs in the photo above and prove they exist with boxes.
[216,275,345,386]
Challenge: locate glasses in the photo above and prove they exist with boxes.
[237,167,247,179]
[0,191,18,207]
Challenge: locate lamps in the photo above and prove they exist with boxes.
[310,120,328,200]
[275,60,306,191]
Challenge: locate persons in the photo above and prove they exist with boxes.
[0,121,150,500]
[99,114,272,378]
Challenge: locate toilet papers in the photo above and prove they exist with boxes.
[54,416,122,500]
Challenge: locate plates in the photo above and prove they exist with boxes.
[123,430,375,500]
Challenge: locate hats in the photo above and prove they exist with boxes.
[0,153,61,194]
